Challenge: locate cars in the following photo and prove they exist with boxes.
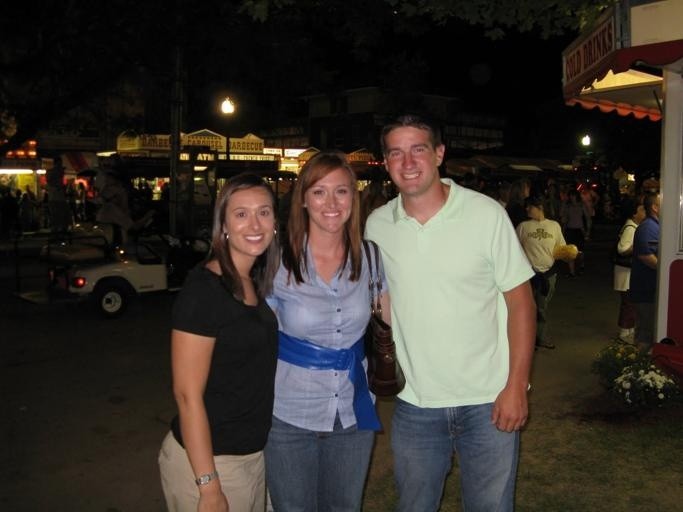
[40,234,214,319]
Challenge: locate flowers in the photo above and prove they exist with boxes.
[595,334,681,412]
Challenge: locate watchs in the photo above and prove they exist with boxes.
[195,472,221,485]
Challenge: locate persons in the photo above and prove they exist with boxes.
[494,180,660,351]
[366,114,540,512]
[159,174,279,512]
[244,155,392,512]
[0,157,155,252]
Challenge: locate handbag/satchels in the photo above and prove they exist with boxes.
[612,224,638,268]
[363,240,406,397]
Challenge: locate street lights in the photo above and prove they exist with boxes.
[221,97,235,160]
[581,135,591,191]
